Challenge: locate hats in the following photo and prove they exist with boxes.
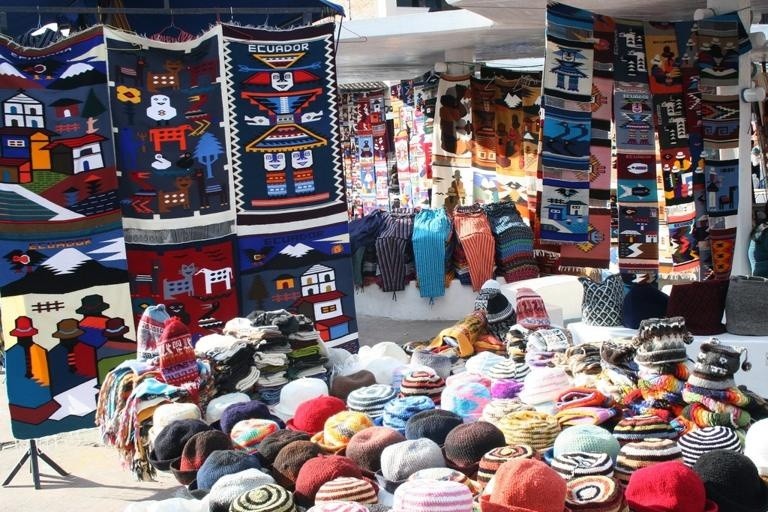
[52,317,85,339]
[76,294,110,315]
[138,304,201,412]
[605,317,754,512]
[406,308,602,374]
[515,286,552,329]
[10,316,39,337]
[145,349,619,511]
[474,279,516,331]
[103,317,130,336]
